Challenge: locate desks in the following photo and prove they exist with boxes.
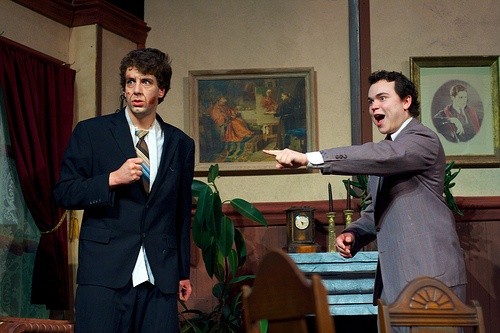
[287,251,379,315]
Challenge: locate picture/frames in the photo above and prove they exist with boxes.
[188,67,321,176]
[409,56,500,168]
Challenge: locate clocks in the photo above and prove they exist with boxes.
[285,206,320,253]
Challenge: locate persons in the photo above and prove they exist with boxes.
[263,71,468,333]
[54,48,195,333]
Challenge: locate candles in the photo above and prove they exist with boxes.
[347,179,351,210]
[328,183,334,213]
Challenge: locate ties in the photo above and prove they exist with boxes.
[135,129,149,196]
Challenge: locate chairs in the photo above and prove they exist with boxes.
[378,277,485,333]
[241,249,335,333]
